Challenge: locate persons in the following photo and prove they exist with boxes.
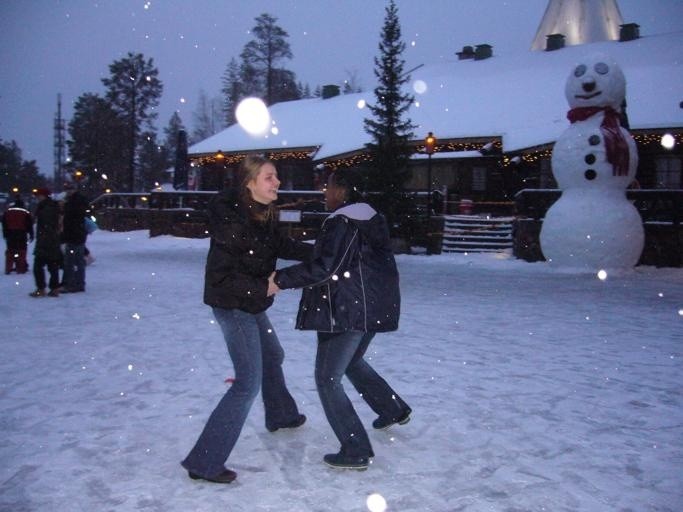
[0,181,97,298]
[267,163,412,471]
[180,152,315,485]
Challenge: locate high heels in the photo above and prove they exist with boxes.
[323,451,370,468]
[373,414,410,429]
[265,415,306,432]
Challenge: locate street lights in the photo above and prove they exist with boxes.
[216,150,226,193]
[424,132,435,230]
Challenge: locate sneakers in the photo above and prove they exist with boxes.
[5,269,86,297]
[189,468,237,484]
